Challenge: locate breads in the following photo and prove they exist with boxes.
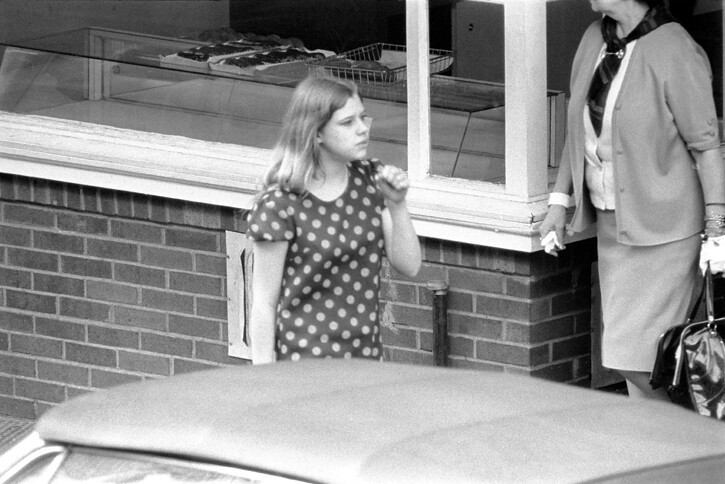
[175,27,397,82]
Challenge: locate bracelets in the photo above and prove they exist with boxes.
[704,212,725,237]
[548,192,570,209]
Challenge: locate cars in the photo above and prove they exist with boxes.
[1,359,725,484]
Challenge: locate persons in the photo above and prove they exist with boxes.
[244,74,421,366]
[538,0,725,401]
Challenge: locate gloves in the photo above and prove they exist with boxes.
[698,234,725,276]
[539,232,567,256]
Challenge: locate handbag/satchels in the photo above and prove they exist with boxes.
[648,262,725,422]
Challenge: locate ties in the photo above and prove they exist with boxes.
[587,5,666,138]
